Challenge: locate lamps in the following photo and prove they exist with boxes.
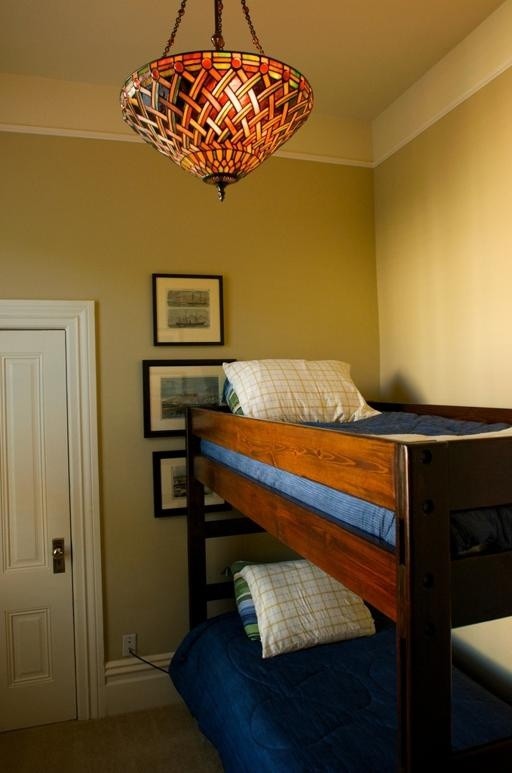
[113,0,319,200]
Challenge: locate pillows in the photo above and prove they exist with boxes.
[221,356,382,424]
[228,554,380,660]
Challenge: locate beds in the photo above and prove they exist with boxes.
[179,400,512,770]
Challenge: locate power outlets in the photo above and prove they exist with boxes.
[123,633,138,655]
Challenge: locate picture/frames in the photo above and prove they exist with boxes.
[141,274,237,518]
